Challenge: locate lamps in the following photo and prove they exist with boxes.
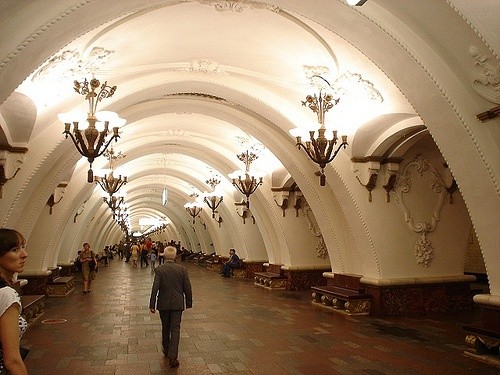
[184,192,202,224]
[288,87,349,187]
[93,146,129,202]
[227,149,264,209]
[102,192,131,236]
[56,74,127,183]
[143,220,170,236]
[195,173,226,218]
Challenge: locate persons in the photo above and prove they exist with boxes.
[149,245,193,368]
[219,249,240,277]
[0,228,29,375]
[80,243,97,293]
[103,234,191,272]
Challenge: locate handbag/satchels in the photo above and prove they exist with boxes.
[89,260,95,268]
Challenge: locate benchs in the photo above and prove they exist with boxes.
[461,304,500,368]
[229,259,247,279]
[44,266,75,297]
[309,275,374,316]
[181,251,220,272]
[12,281,45,328]
[253,264,287,291]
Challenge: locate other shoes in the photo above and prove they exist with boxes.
[163,347,168,356]
[83,291,87,294]
[88,288,90,293]
[168,359,179,366]
